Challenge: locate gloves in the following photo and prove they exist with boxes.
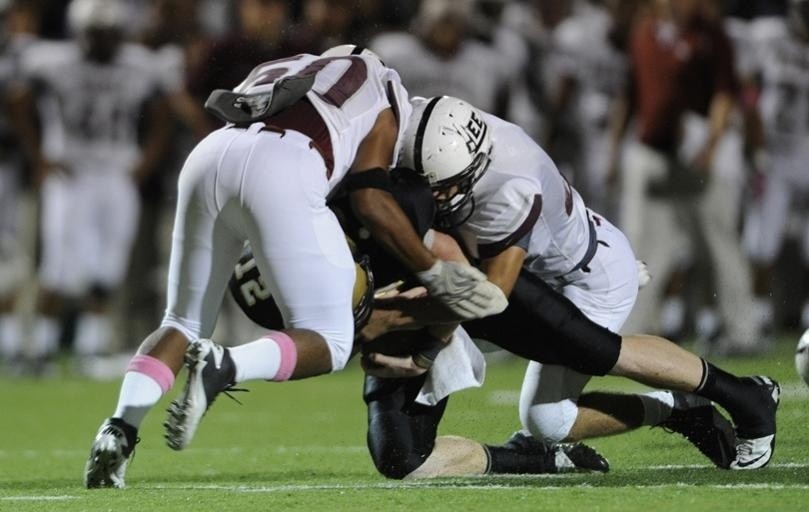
[416,257,496,320]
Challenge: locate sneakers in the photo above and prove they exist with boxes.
[665,391,738,470]
[85,419,141,490]
[730,375,781,472]
[507,428,609,474]
[164,338,249,450]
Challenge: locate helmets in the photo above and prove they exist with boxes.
[347,236,376,332]
[396,94,494,232]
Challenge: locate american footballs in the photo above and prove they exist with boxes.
[356,272,462,370]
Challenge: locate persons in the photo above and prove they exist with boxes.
[1,1,809,380]
[394,92,737,469]
[227,165,783,481]
[84,43,413,493]
